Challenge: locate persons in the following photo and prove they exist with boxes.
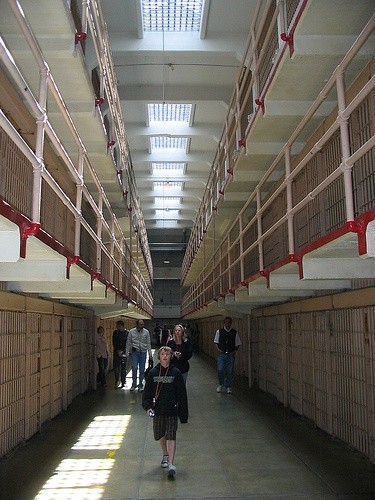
[112,321,129,389]
[95,326,110,387]
[141,346,189,476]
[215,316,243,394]
[166,324,193,388]
[126,319,153,393]
[155,320,198,349]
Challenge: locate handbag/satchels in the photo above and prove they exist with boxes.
[145,362,153,380]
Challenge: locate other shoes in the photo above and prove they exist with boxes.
[130,386,135,391]
[138,387,144,392]
[161,454,169,467]
[166,464,176,477]
[115,381,118,385]
[122,382,124,387]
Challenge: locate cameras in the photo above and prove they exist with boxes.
[150,411,155,416]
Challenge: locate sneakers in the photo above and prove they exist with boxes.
[226,386,232,393]
[216,384,223,392]
